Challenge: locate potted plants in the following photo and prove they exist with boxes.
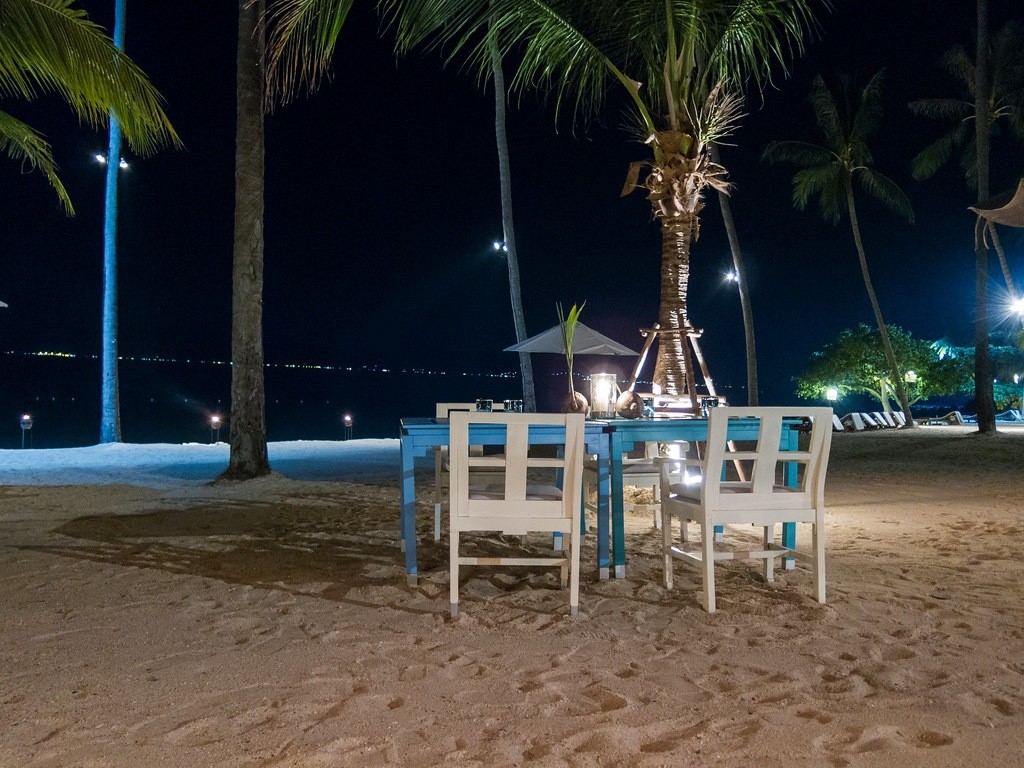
[555,298,589,417]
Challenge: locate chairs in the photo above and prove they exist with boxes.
[449,410,585,619]
[653,406,834,613]
[585,394,689,543]
[433,402,523,546]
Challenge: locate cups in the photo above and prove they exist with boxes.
[639,397,654,418]
[503,400,522,413]
[476,399,493,412]
[701,397,719,418]
[590,374,617,419]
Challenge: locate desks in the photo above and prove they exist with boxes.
[399,417,610,589]
[587,418,803,578]
